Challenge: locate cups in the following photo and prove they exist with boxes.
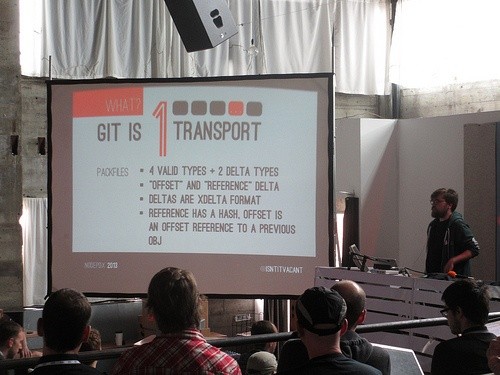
[115,333,123,346]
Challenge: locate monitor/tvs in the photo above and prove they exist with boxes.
[349,244,370,273]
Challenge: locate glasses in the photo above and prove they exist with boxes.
[440,307,451,318]
[430,200,444,205]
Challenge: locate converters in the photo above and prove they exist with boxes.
[374,263,392,270]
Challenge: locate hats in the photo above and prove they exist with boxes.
[246,351,278,371]
[296,286,347,336]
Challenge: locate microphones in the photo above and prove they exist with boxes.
[448,271,474,280]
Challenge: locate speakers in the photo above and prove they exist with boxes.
[164,0,239,54]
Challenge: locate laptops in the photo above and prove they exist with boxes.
[374,258,398,271]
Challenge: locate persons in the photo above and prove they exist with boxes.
[430,279,500,375]
[425,187,480,281]
[0,267,390,375]
